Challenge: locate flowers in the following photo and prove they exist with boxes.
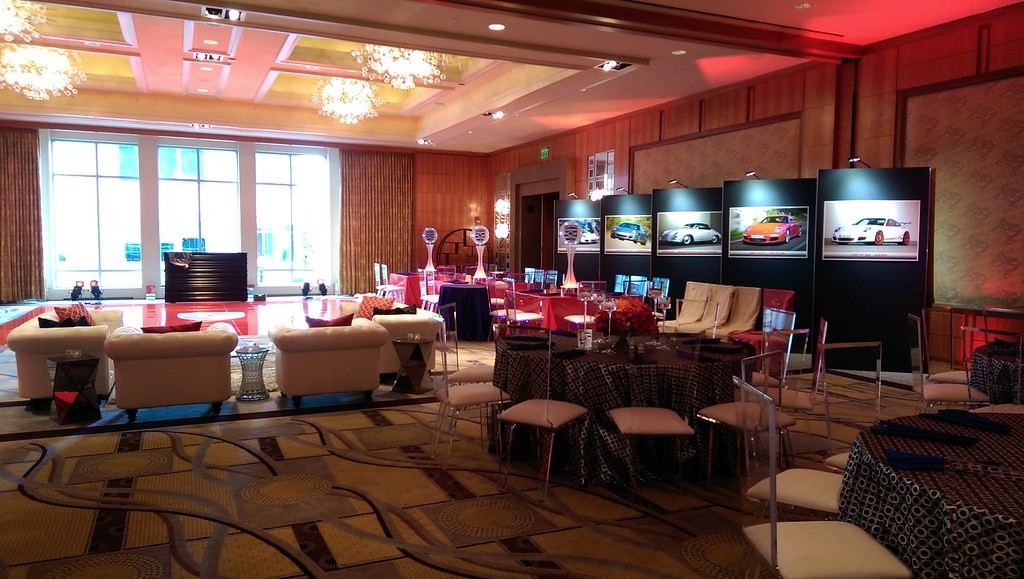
[592,295,657,335]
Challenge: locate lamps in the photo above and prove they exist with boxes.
[422,227,438,275]
[0,0,87,102]
[603,59,621,73]
[667,179,689,189]
[559,221,583,295]
[308,76,389,125]
[470,226,489,285]
[351,43,461,92]
[567,192,579,199]
[745,170,761,180]
[848,156,871,169]
[615,187,631,195]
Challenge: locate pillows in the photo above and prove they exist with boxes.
[354,296,395,321]
[305,313,355,328]
[37,316,74,328]
[54,302,96,326]
[64,316,90,328]
[140,321,203,334]
[372,304,417,316]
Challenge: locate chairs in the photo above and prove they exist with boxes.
[908,313,989,415]
[705,286,761,342]
[606,343,702,506]
[657,280,710,334]
[417,263,648,336]
[648,277,670,320]
[698,352,799,496]
[741,350,844,569]
[491,323,589,503]
[922,307,971,411]
[820,342,883,475]
[374,263,398,302]
[751,307,797,388]
[733,318,829,457]
[676,283,734,334]
[962,326,1024,414]
[381,264,405,305]
[418,318,512,472]
[729,288,794,374]
[437,303,496,442]
[732,377,913,579]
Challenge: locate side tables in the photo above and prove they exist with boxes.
[393,333,433,395]
[235,342,270,403]
[46,350,102,424]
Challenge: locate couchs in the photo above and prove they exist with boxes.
[339,295,444,383]
[102,320,239,422]
[5,302,124,405]
[267,312,389,408]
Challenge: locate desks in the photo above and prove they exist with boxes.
[388,272,467,309]
[492,330,755,490]
[970,341,1024,405]
[836,413,1024,579]
[504,288,645,332]
[434,279,540,323]
